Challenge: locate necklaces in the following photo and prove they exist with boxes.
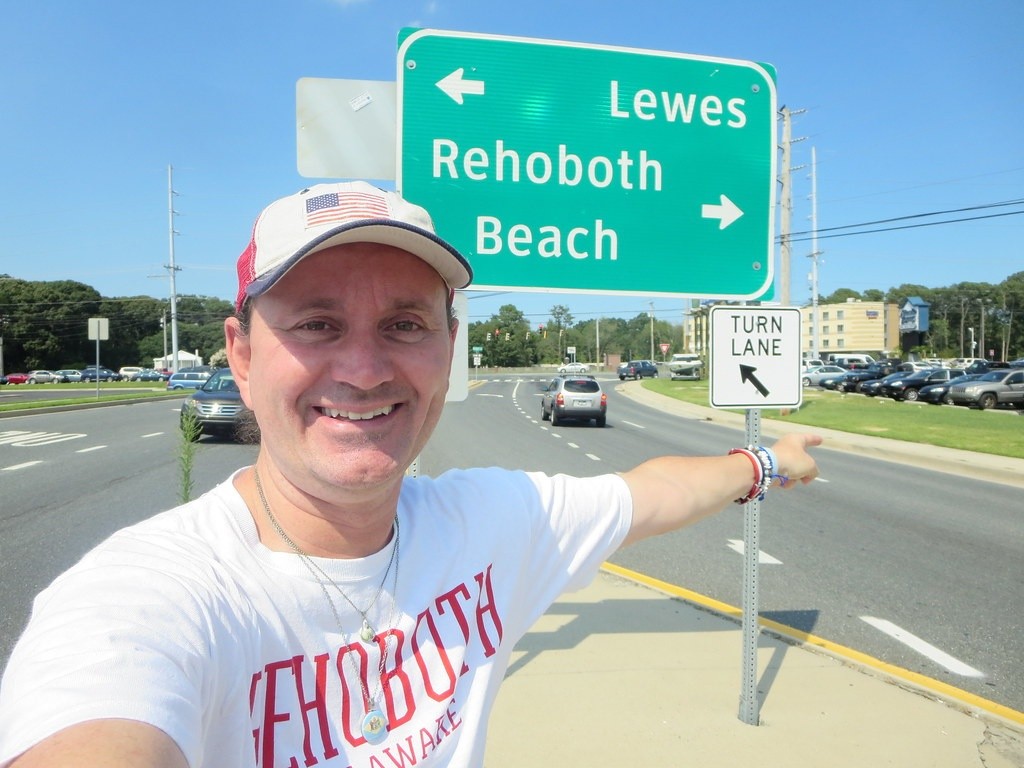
[252,465,399,744]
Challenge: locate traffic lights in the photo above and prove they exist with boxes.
[505,332,510,343]
[486,331,491,342]
[539,324,542,334]
[526,331,530,340]
[559,329,564,338]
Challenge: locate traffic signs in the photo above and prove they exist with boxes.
[395,26,779,303]
[710,303,803,410]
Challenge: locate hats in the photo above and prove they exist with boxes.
[234,181,472,314]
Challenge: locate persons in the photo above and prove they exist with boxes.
[0,180,825,767]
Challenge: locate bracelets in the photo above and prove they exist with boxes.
[732,443,789,505]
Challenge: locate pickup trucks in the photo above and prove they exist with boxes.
[617,360,658,380]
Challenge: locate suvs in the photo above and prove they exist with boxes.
[880,368,972,402]
[541,374,607,427]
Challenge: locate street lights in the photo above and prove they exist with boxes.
[968,327,974,358]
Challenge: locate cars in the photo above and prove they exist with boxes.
[56,370,83,382]
[916,374,985,406]
[557,363,590,374]
[81,368,122,383]
[119,365,229,391]
[5,373,29,384]
[949,369,1024,408]
[28,369,65,385]
[802,352,1023,392]
[180,368,247,444]
[0,375,7,385]
[857,371,912,397]
